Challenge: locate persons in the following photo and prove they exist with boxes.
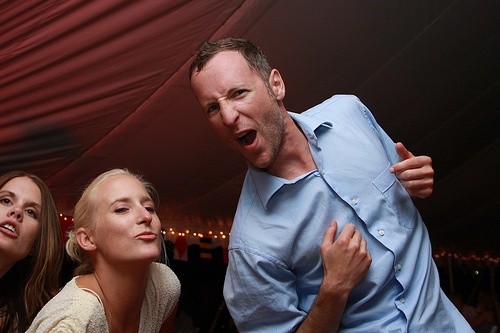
[24,169,184,333]
[188,36,479,333]
[0,170,64,333]
